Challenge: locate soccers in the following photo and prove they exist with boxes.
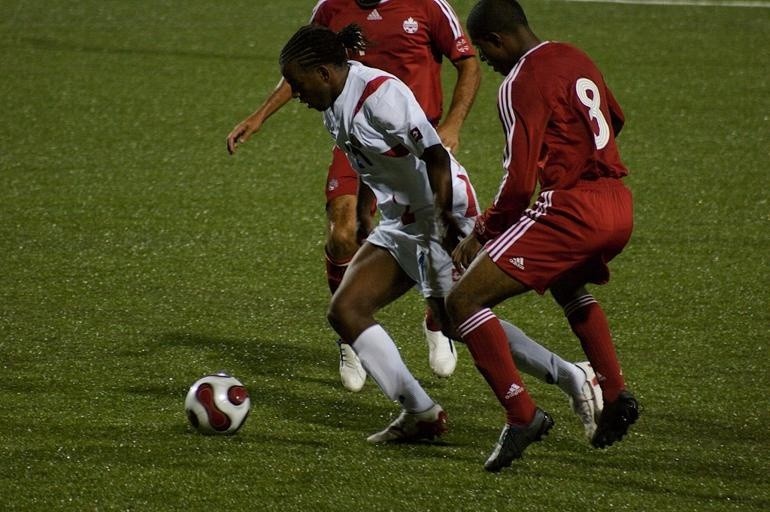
[185,373,251,435]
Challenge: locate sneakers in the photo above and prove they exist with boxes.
[423,311,457,377]
[336,341,368,390]
[570,361,602,439]
[368,405,447,445]
[592,390,637,448]
[484,408,553,471]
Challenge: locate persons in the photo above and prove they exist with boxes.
[278,25,604,442]
[227,1,481,392]
[445,0,641,471]
